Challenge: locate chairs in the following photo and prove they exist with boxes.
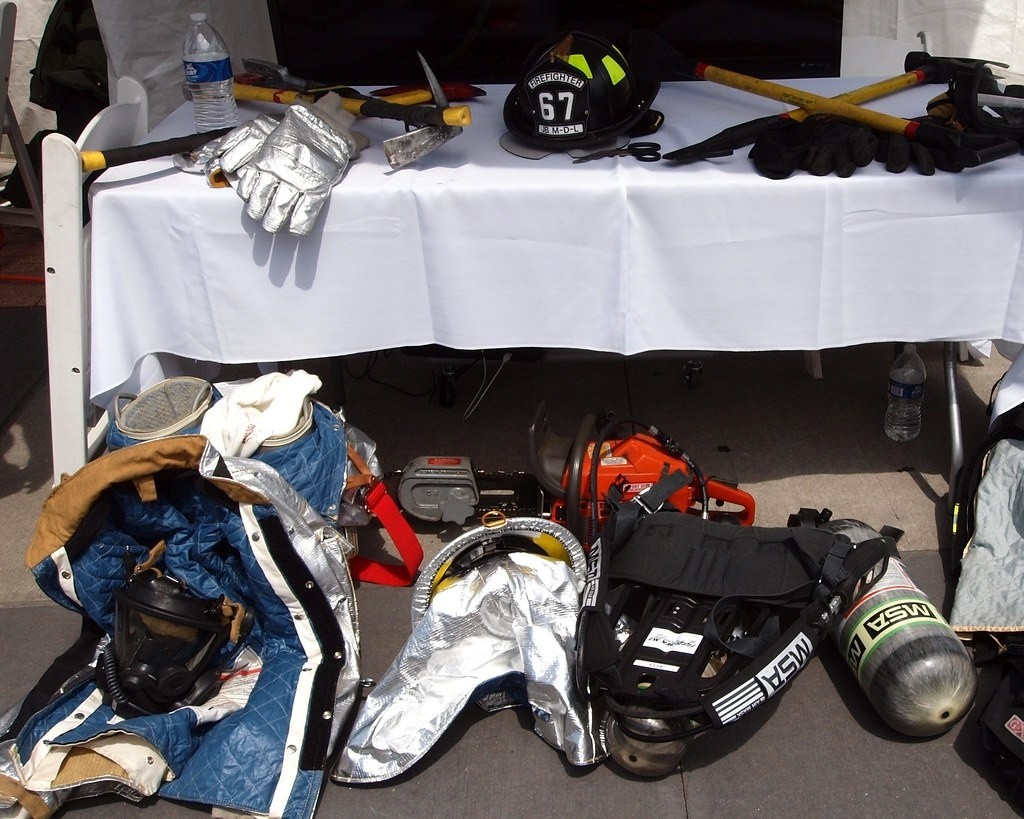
[0,60,185,490]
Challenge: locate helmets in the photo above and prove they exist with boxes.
[503,27,661,149]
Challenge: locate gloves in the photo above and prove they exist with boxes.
[173,113,369,174]
[746,64,1024,180]
[237,98,357,234]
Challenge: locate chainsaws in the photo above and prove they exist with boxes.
[383,399,755,536]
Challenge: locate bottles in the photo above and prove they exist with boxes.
[182,12,239,133]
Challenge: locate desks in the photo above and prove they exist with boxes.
[90,76,1024,513]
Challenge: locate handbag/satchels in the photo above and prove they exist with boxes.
[981,347,1024,442]
[106,368,426,588]
[582,502,905,742]
[948,444,1009,662]
[0,432,366,819]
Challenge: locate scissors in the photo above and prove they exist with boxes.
[601,142,662,163]
